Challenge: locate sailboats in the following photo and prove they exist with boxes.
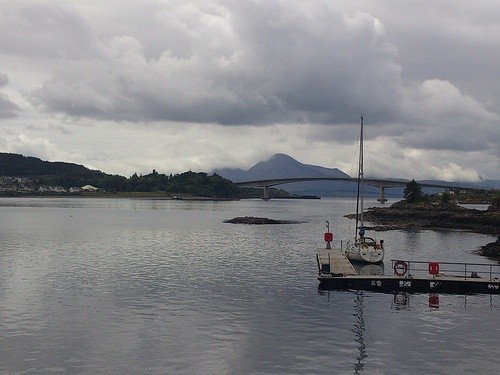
[344,113,383,264]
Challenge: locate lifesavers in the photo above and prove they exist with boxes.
[394,261,407,276]
[362,243,368,249]
